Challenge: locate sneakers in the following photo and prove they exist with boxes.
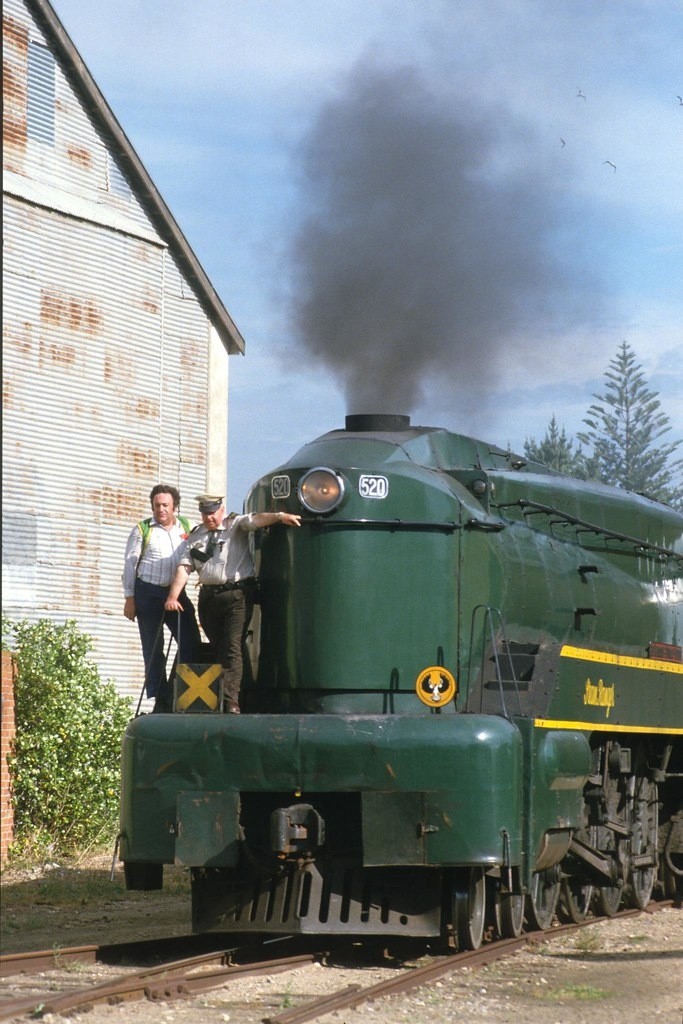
[226,701,240,714]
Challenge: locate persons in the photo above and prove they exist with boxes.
[121,483,203,714]
[165,493,303,712]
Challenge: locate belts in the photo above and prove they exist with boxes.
[207,579,255,592]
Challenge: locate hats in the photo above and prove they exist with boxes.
[195,495,225,513]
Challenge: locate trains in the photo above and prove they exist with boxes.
[106,409,681,969]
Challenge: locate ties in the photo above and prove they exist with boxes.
[190,530,221,562]
